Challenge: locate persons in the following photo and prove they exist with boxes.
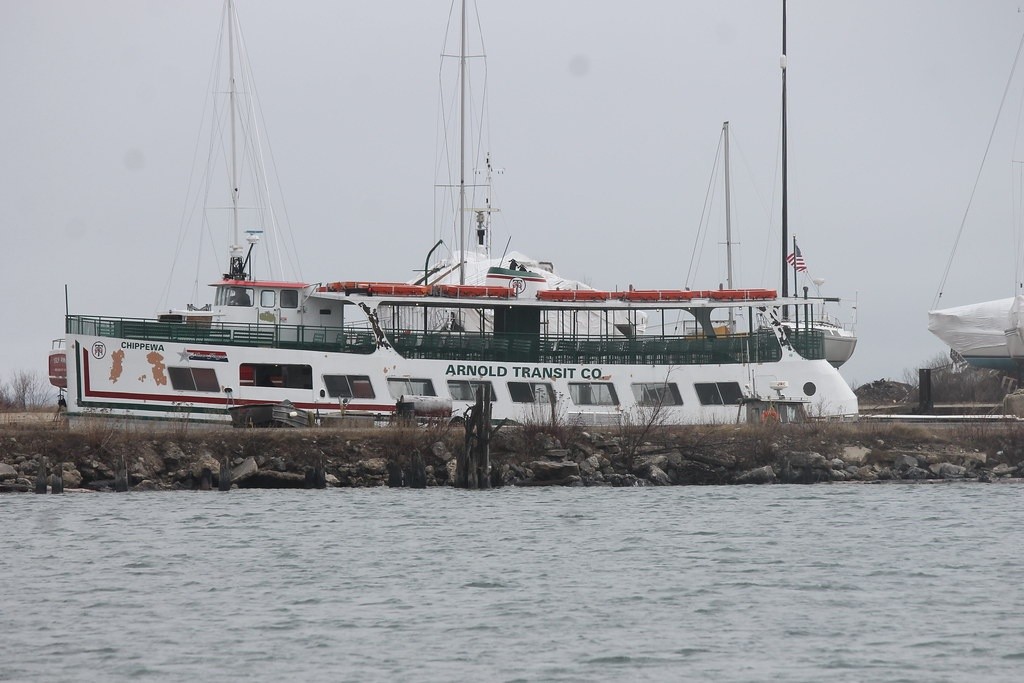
[229,289,251,306]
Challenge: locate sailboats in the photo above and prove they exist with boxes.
[928,35,1023,382]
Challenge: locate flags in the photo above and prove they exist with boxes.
[787,246,807,272]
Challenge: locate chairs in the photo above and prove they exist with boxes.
[311,332,821,363]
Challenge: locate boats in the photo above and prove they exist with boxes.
[48,0,862,430]
[227,402,310,430]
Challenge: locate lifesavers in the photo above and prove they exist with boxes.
[761,410,777,423]
